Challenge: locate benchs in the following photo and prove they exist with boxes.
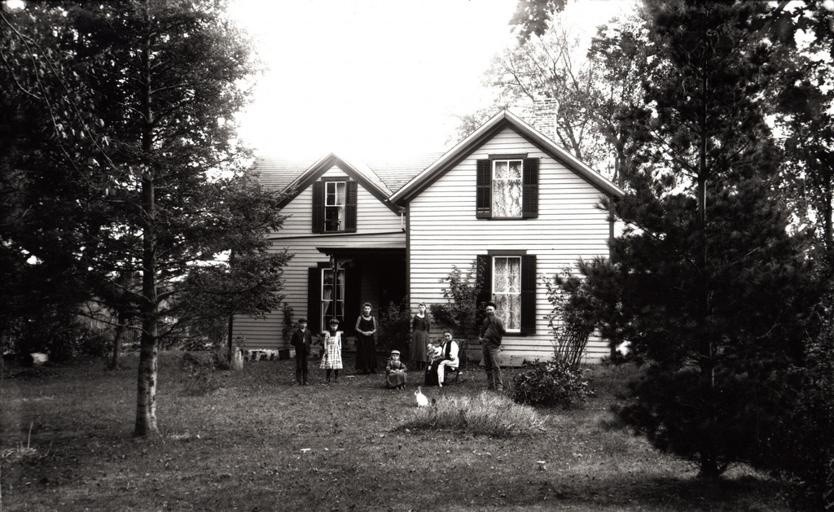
[435,340,465,384]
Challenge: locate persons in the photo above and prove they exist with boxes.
[423,338,443,386]
[321,318,344,384]
[411,302,431,372]
[291,318,311,385]
[435,331,460,387]
[478,301,507,391]
[354,302,379,374]
[385,349,407,390]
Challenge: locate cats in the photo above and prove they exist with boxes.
[414,386,431,408]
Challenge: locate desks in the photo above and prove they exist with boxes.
[249,348,280,361]
